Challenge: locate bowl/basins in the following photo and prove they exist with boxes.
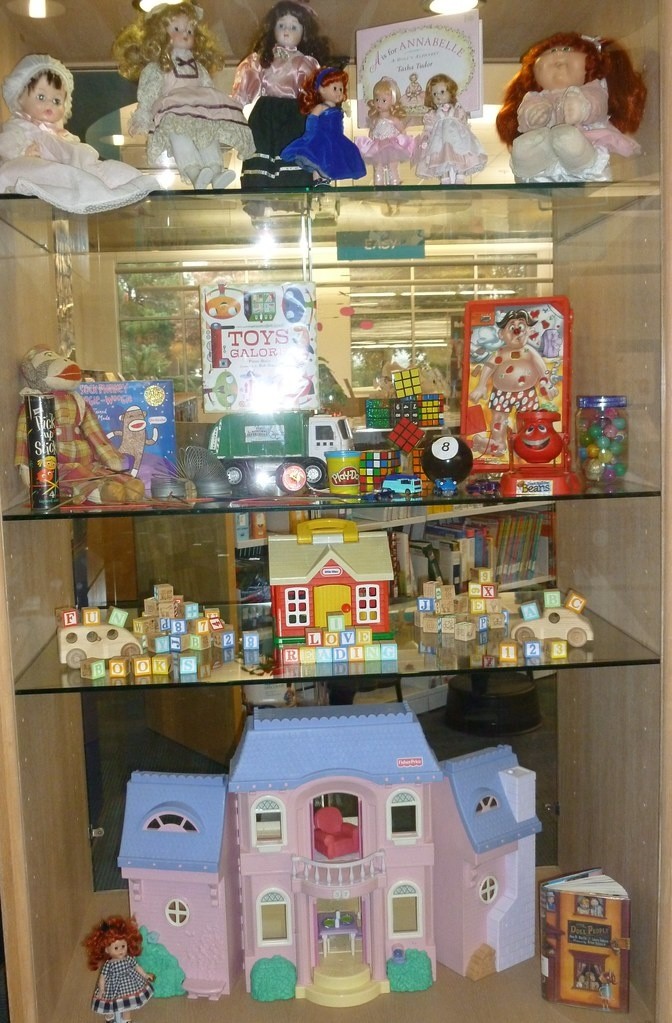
[442,672,544,738]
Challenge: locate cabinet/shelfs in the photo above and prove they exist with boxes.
[131,398,559,768]
[0,0,672,1023]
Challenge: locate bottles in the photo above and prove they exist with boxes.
[575,394,628,483]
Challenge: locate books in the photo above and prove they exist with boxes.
[236,509,352,541]
[540,868,631,1014]
[355,8,483,128]
[199,281,320,413]
[352,506,555,599]
[79,380,178,489]
[401,677,448,689]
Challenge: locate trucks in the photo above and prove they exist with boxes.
[208,412,357,490]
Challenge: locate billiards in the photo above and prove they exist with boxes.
[421,434,473,484]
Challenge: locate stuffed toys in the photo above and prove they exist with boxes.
[14,345,143,504]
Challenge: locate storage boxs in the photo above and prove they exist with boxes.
[460,294,575,478]
[198,280,321,415]
[207,413,307,460]
[76,381,179,500]
[335,229,426,261]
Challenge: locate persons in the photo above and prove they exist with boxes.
[82,915,155,1023]
[495,32,647,182]
[0,54,159,215]
[114,2,256,190]
[284,683,294,705]
[354,76,415,185]
[231,0,330,187]
[281,67,367,186]
[416,74,488,184]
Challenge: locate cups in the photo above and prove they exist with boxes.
[324,450,361,497]
